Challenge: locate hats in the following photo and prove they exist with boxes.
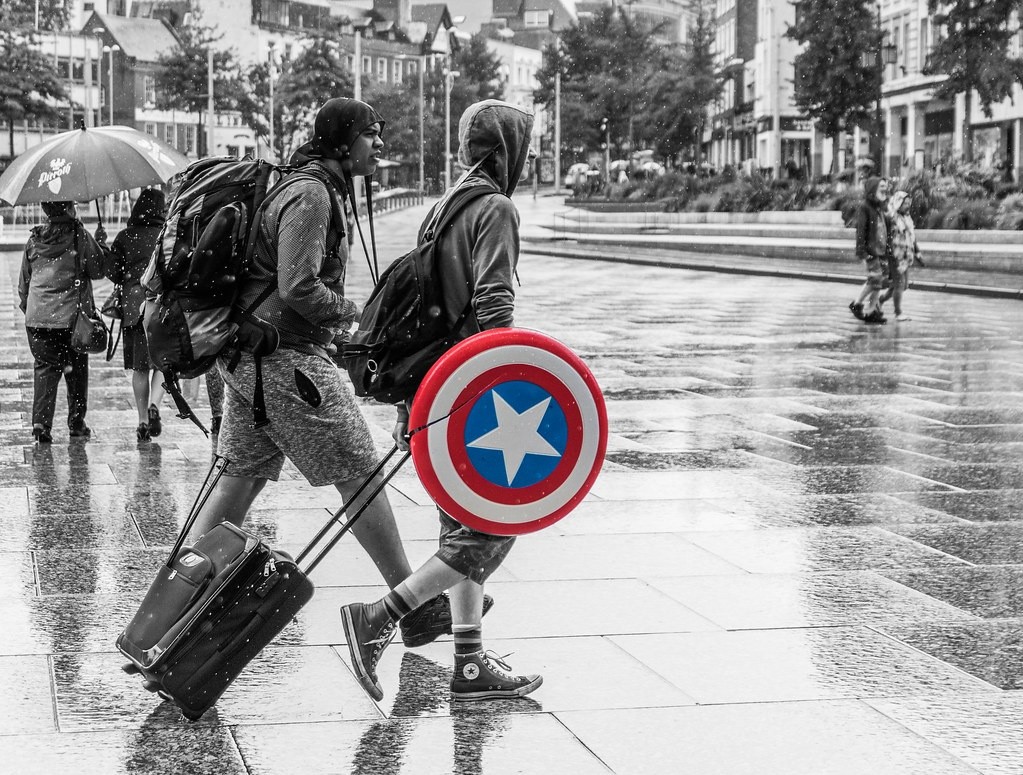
[289,97,384,167]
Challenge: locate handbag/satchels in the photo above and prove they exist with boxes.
[101,255,124,319]
[72,308,106,354]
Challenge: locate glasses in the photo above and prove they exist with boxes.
[71,202,78,208]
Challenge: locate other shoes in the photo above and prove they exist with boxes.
[896,314,911,321]
[865,311,887,324]
[849,302,865,319]
[33,428,52,442]
[69,427,90,436]
[876,302,883,314]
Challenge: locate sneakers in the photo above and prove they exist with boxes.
[399,592,494,647]
[450,649,543,702]
[340,599,397,702]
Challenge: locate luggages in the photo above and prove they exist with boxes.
[116,440,412,722]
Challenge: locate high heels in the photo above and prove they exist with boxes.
[137,423,150,441]
[148,404,161,437]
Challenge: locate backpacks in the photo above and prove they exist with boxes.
[342,186,503,404]
[139,155,353,379]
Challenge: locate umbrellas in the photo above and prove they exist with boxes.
[0,119,191,227]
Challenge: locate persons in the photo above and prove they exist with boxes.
[19,200,110,441]
[189,96,496,647]
[102,185,224,440]
[340,100,543,702]
[846,176,925,324]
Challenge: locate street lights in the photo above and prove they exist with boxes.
[105,44,121,127]
[441,68,461,191]
[397,54,426,191]
